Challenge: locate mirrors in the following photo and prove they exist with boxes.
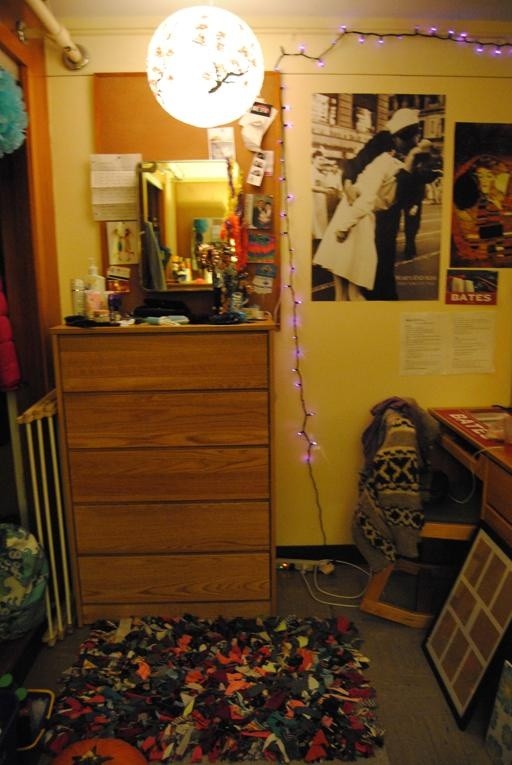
[153,162,240,284]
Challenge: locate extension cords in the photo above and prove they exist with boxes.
[275,557,335,575]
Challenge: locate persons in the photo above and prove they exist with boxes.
[341,108,438,301]
[312,131,422,302]
[253,200,272,228]
[259,202,272,228]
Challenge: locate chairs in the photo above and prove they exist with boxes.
[359,394,478,630]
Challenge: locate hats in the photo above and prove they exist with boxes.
[386,108,420,134]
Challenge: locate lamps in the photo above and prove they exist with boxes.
[145,3,266,129]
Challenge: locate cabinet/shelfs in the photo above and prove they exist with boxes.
[51,306,279,625]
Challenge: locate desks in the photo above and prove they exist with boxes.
[429,407,512,550]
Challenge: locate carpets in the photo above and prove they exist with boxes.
[33,617,388,765]
